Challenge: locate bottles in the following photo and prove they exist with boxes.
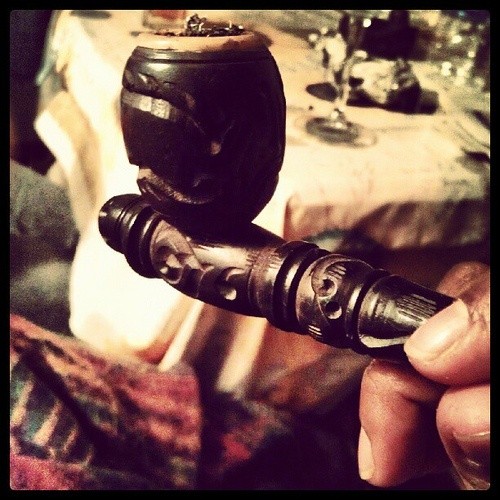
[307,8,382,142]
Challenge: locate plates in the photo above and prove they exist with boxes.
[456,99,490,147]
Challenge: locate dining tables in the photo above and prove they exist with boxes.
[28,7,497,418]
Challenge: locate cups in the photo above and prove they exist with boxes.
[425,10,483,90]
[141,10,186,29]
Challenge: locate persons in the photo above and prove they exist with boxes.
[359,265,492,493]
